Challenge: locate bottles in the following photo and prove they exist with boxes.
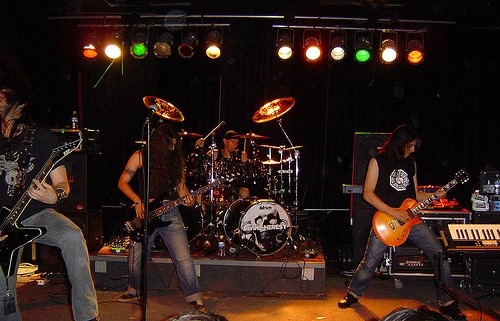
[217,235,226,257]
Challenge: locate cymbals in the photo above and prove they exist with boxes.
[252,97,295,123]
[231,133,271,139]
[262,160,279,164]
[182,133,204,137]
[142,96,186,123]
[258,145,280,149]
[286,146,303,150]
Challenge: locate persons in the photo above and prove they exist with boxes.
[195,130,250,197]
[0,83,101,321]
[118,120,208,312]
[338,124,467,321]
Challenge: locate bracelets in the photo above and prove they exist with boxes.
[54,188,67,204]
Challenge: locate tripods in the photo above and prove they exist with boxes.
[188,121,231,247]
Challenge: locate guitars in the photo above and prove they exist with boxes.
[372,169,470,247]
[0,139,84,278]
[123,177,227,243]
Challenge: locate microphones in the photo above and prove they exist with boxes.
[145,104,157,124]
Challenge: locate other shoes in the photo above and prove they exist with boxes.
[119,291,140,301]
[338,293,358,309]
[189,301,208,311]
[439,304,467,321]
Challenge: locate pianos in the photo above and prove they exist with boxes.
[436,221,500,254]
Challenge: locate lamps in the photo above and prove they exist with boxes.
[275,27,295,60]
[377,30,399,64]
[327,28,347,62]
[130,27,150,59]
[302,28,324,62]
[178,25,200,59]
[204,26,224,60]
[80,25,102,61]
[153,26,174,58]
[405,32,425,65]
[353,30,374,64]
[104,27,125,59]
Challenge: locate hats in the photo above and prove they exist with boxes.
[224,130,239,140]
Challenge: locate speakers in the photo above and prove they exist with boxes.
[35,154,104,274]
[471,211,500,284]
[349,132,394,272]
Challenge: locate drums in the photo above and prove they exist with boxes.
[243,159,265,185]
[203,199,231,239]
[178,202,206,243]
[224,195,292,257]
[186,151,213,190]
[217,156,244,186]
